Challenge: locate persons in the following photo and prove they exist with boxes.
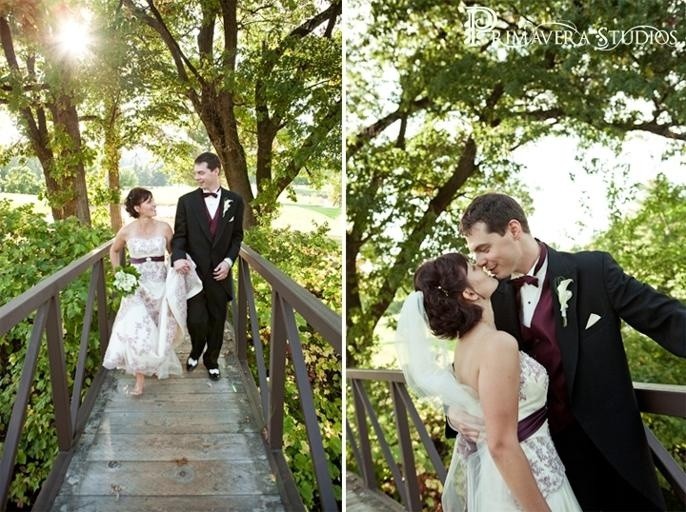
[445,194,686,512]
[170,152,244,380]
[109,187,191,395]
[413,253,584,511]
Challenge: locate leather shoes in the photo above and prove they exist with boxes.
[208,367,219,381]
[187,357,198,371]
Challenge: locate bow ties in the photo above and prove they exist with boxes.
[202,186,221,197]
[512,242,546,288]
